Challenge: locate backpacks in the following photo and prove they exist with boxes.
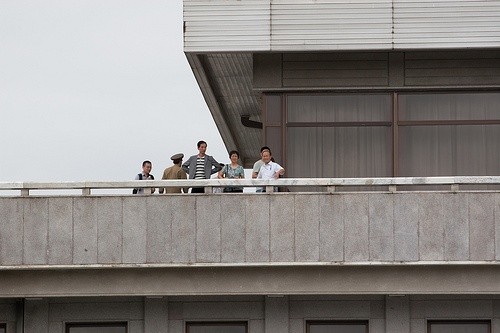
[133,174,153,194]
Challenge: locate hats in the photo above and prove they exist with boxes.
[171,154,184,161]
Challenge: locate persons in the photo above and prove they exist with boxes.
[159,154,188,193]
[218,151,244,193]
[256,150,285,192]
[251,146,270,178]
[182,140,222,193]
[210,163,225,193]
[133,160,155,194]
